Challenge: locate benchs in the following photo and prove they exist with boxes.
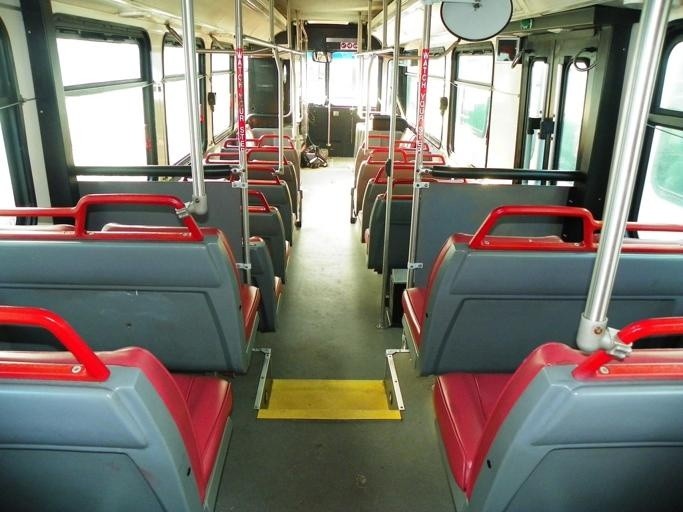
[181,135,301,334]
[398,204,682,378]
[1,306,235,511]
[1,192,262,379]
[430,316,683,510]
[352,132,466,276]
